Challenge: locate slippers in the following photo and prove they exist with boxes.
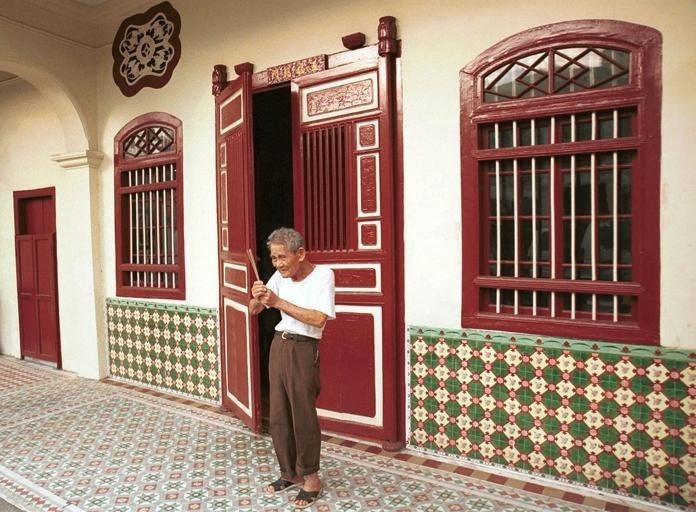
[295,487,322,508]
[268,478,303,493]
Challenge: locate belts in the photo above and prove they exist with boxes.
[275,331,309,341]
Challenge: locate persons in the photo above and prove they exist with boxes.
[248,226,337,509]
